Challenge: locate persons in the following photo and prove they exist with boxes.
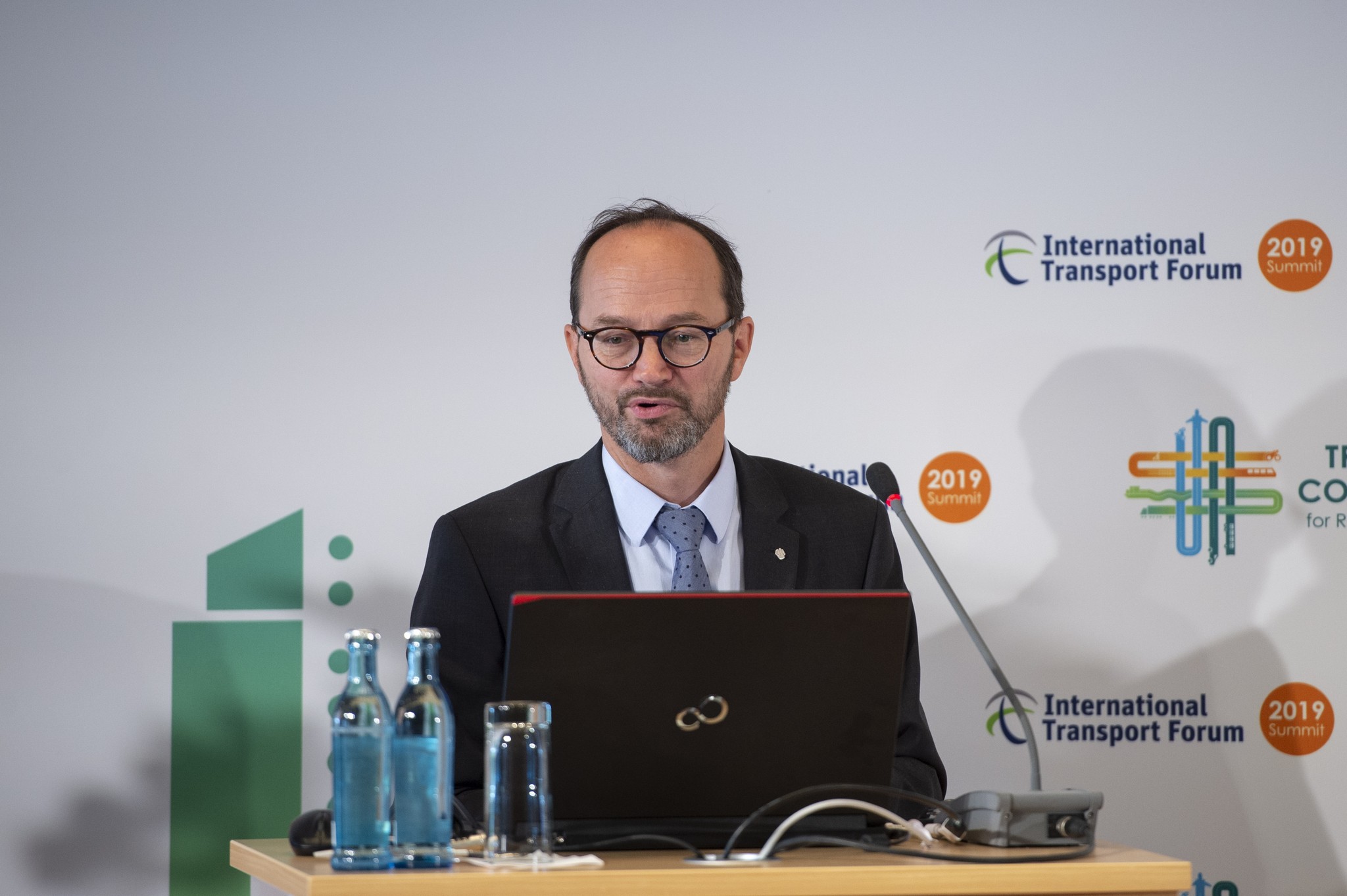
[408,198,948,839]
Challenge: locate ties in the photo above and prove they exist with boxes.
[651,507,715,592]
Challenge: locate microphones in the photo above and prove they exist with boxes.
[864,462,1105,848]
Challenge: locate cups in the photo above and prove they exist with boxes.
[483,700,552,861]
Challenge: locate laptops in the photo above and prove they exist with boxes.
[499,592,906,849]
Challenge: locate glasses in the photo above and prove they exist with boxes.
[572,311,741,370]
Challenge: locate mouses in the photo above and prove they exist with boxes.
[288,808,330,856]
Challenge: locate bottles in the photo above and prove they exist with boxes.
[331,628,392,870]
[392,627,456,869]
